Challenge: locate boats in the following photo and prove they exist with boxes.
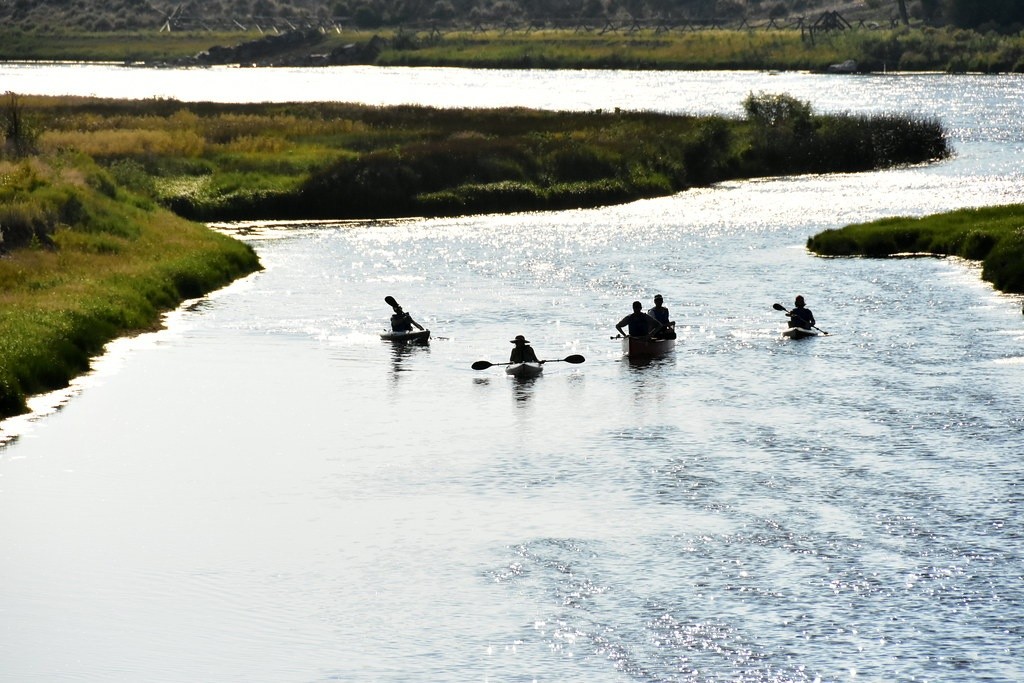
[783,327,819,341]
[380,328,431,342]
[618,328,679,360]
[504,362,543,379]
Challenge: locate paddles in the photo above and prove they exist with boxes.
[385,295,410,316]
[610,332,677,339]
[773,302,829,335]
[472,353,586,370]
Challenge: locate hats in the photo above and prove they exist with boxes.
[510,336,530,343]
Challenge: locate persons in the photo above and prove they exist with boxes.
[615,294,670,338]
[509,335,539,364]
[785,295,816,330]
[390,305,414,332]
